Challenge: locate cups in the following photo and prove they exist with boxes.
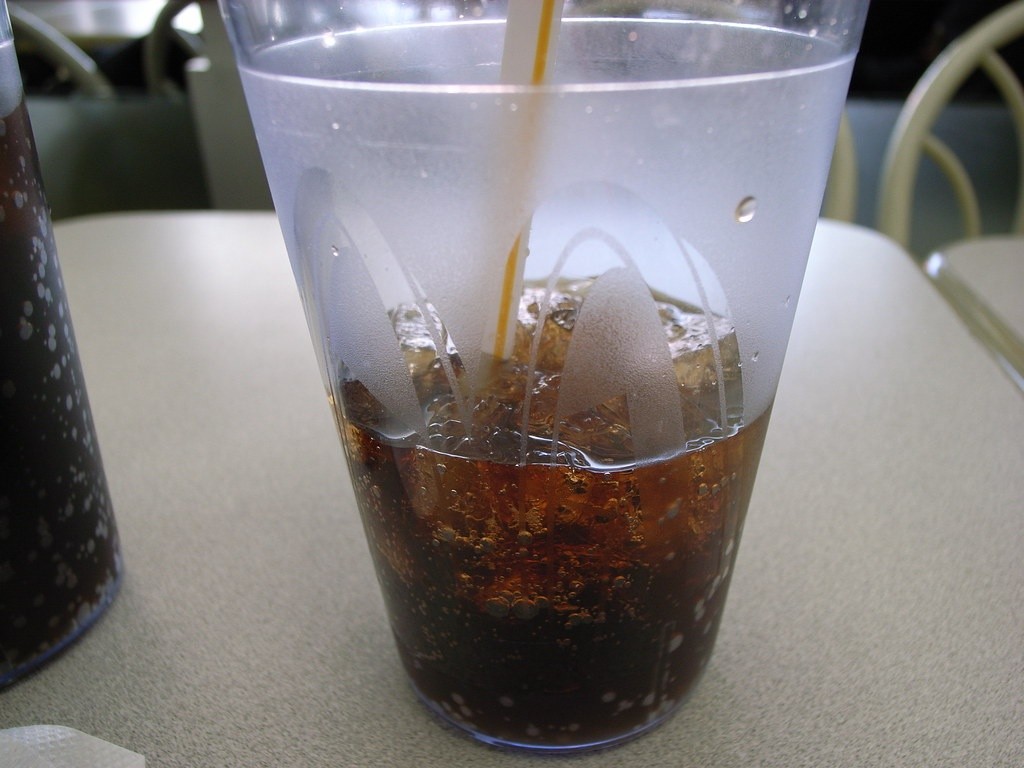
[0,0,124,691]
[217,0,872,754]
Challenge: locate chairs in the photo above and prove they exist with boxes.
[881,0,1024,258]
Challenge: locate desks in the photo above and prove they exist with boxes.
[0,212,1023,767]
[921,235,1024,374]
